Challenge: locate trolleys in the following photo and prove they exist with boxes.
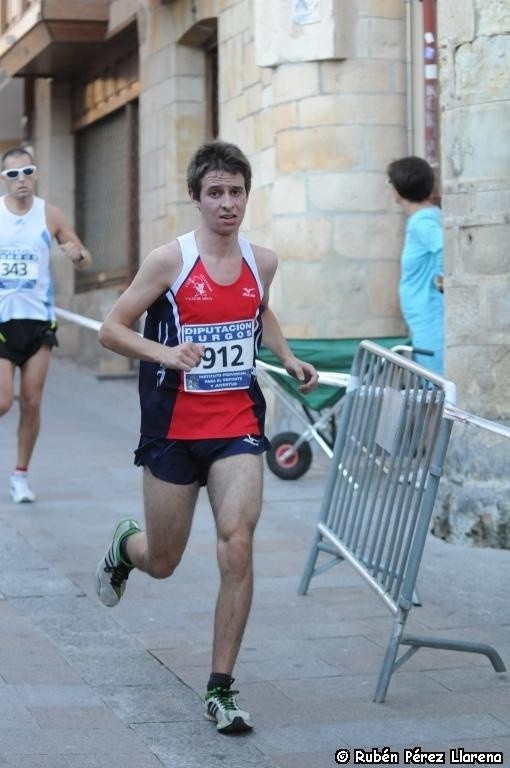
[254,334,442,490]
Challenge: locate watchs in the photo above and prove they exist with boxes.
[73,248,86,264]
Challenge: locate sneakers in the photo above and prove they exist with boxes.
[397,468,445,489]
[204,687,253,731]
[95,518,141,606]
[10,477,35,503]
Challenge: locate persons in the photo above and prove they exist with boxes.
[0,148,92,504]
[94,142,319,736]
[387,156,445,486]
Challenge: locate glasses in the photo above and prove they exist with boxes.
[2,166,36,179]
